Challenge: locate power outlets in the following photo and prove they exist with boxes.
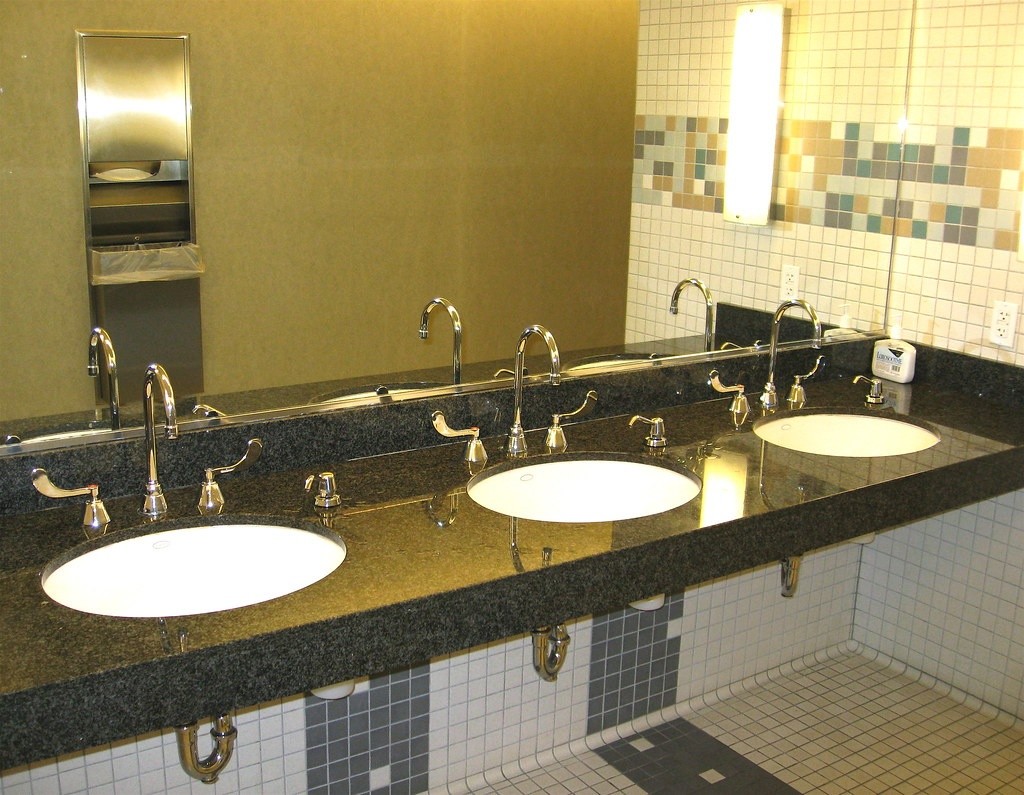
[779,263,800,301]
[987,300,1019,349]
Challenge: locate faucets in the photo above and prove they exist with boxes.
[141,360,180,516]
[504,324,561,457]
[416,295,464,386]
[86,324,124,431]
[757,299,822,405]
[669,276,717,350]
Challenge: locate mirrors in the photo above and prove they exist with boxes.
[0,0,915,459]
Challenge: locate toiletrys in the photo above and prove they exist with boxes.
[870,313,916,383]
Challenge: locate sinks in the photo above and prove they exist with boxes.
[0,420,157,448]
[37,508,351,621]
[750,397,946,459]
[307,380,456,403]
[561,348,678,373]
[463,441,708,523]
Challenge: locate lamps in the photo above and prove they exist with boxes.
[722,4,783,227]
